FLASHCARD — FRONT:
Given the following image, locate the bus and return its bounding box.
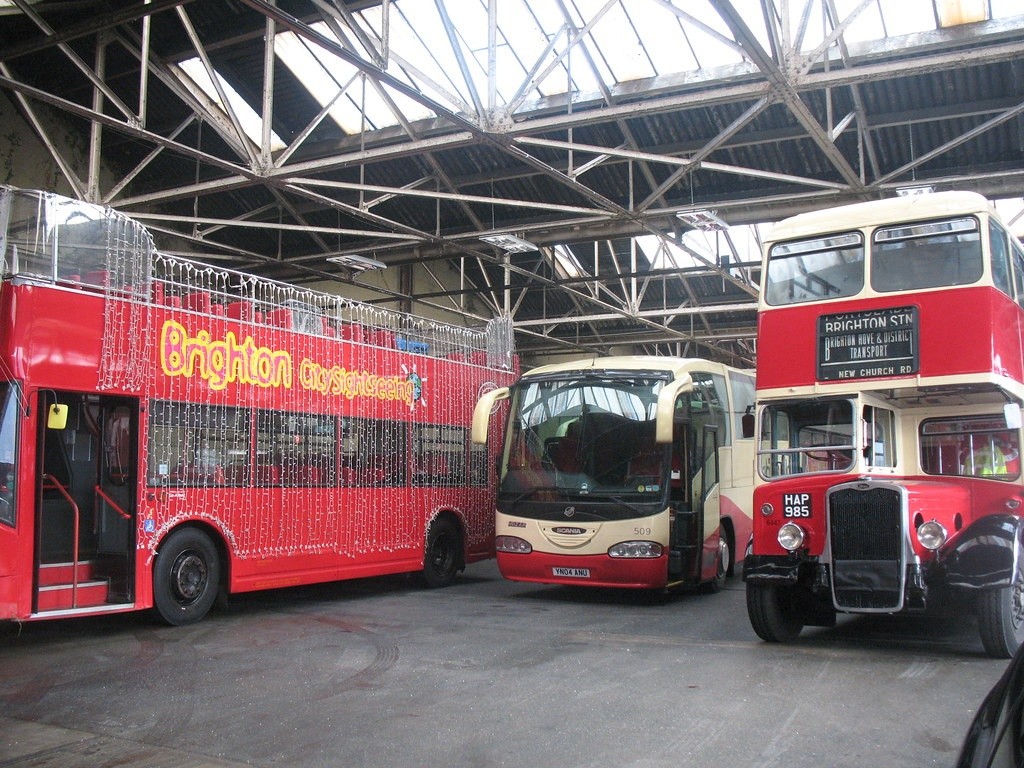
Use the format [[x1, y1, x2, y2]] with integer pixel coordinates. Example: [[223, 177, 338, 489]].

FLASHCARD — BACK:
[[471, 355, 793, 596], [742, 190, 1024, 663], [1, 189, 522, 629]]
